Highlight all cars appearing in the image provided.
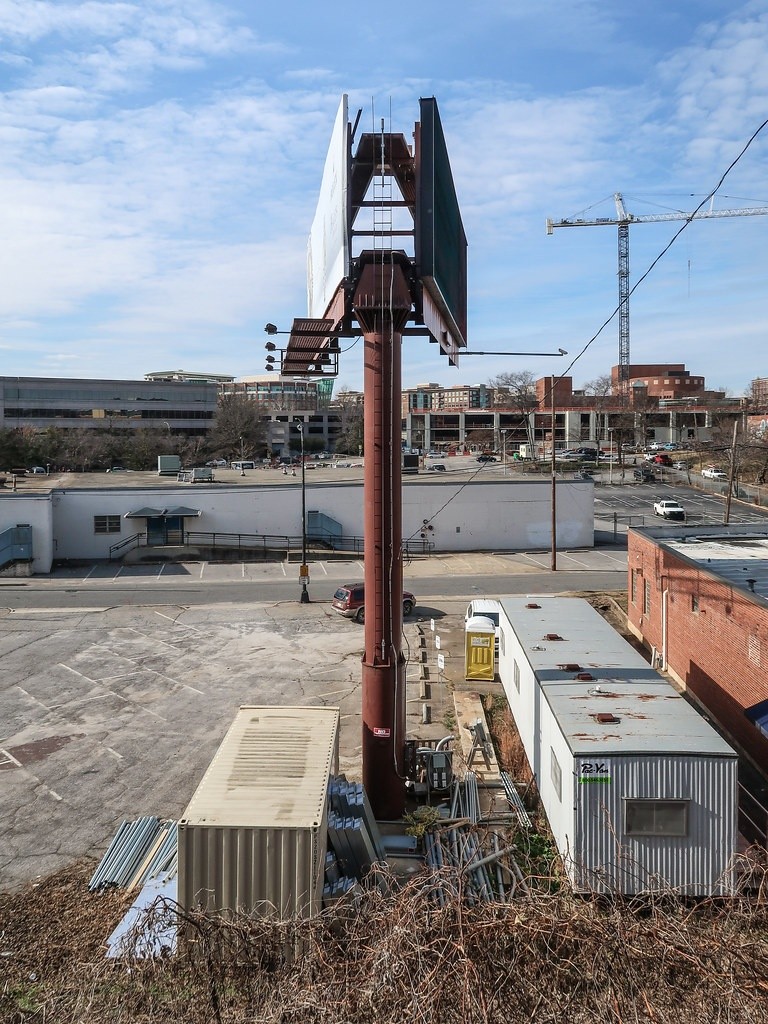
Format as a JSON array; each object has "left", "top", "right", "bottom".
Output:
[
  {"left": 428, "top": 464, "right": 446, "bottom": 471},
  {"left": 477, "top": 456, "right": 496, "bottom": 463},
  {"left": 206, "top": 457, "right": 226, "bottom": 466},
  {"left": 562, "top": 447, "right": 605, "bottom": 459}
]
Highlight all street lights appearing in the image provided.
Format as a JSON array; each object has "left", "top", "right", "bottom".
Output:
[
  {"left": 501, "top": 430, "right": 507, "bottom": 475},
  {"left": 608, "top": 427, "right": 615, "bottom": 484},
  {"left": 418, "top": 430, "right": 425, "bottom": 470},
  {"left": 240, "top": 436, "right": 245, "bottom": 476},
  {"left": 297, "top": 423, "right": 309, "bottom": 603}
]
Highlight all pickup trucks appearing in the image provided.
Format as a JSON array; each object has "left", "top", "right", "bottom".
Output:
[
  {"left": 664, "top": 442, "right": 678, "bottom": 451},
  {"left": 650, "top": 441, "right": 666, "bottom": 450},
  {"left": 655, "top": 454, "right": 672, "bottom": 465},
  {"left": 654, "top": 500, "right": 685, "bottom": 519},
  {"left": 701, "top": 467, "right": 727, "bottom": 481},
  {"left": 644, "top": 452, "right": 658, "bottom": 461}
]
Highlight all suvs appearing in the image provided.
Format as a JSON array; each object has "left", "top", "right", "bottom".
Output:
[
  {"left": 113, "top": 467, "right": 127, "bottom": 472},
  {"left": 620, "top": 442, "right": 631, "bottom": 451},
  {"left": 466, "top": 597, "right": 501, "bottom": 652},
  {"left": 332, "top": 582, "right": 416, "bottom": 624},
  {"left": 426, "top": 452, "right": 446, "bottom": 459},
  {"left": 581, "top": 466, "right": 593, "bottom": 475}
]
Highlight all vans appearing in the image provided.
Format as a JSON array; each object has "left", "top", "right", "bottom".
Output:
[
  {"left": 31, "top": 467, "right": 44, "bottom": 473},
  {"left": 673, "top": 461, "right": 686, "bottom": 470},
  {"left": 280, "top": 457, "right": 302, "bottom": 463}
]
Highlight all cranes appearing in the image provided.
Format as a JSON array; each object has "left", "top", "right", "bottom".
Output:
[{"left": 546, "top": 190, "right": 766, "bottom": 444}]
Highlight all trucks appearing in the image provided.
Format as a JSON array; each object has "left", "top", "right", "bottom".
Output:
[{"left": 634, "top": 469, "right": 655, "bottom": 482}]
